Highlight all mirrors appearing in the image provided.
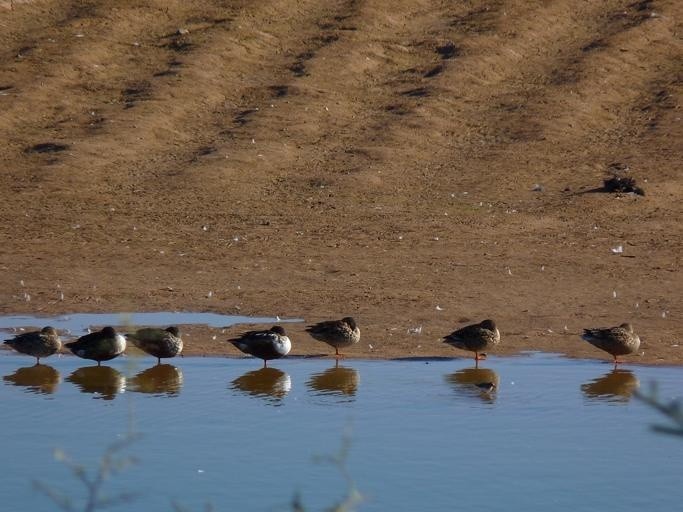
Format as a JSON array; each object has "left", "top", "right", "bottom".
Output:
[
  {"left": 305, "top": 316, "right": 361, "bottom": 356},
  {"left": 224, "top": 360, "right": 291, "bottom": 400},
  {"left": 60, "top": 325, "right": 127, "bottom": 365},
  {"left": 579, "top": 364, "right": 641, "bottom": 403},
  {"left": 226, "top": 324, "right": 293, "bottom": 360},
  {"left": 441, "top": 360, "right": 498, "bottom": 406},
  {"left": 127, "top": 362, "right": 189, "bottom": 398},
  {"left": 63, "top": 367, "right": 125, "bottom": 401},
  {"left": 1, "top": 324, "right": 62, "bottom": 362},
  {"left": 305, "top": 359, "right": 361, "bottom": 399},
  {"left": 2, "top": 364, "right": 60, "bottom": 396},
  {"left": 579, "top": 322, "right": 642, "bottom": 363},
  {"left": 441, "top": 318, "right": 502, "bottom": 361},
  {"left": 124, "top": 325, "right": 184, "bottom": 362}
]
